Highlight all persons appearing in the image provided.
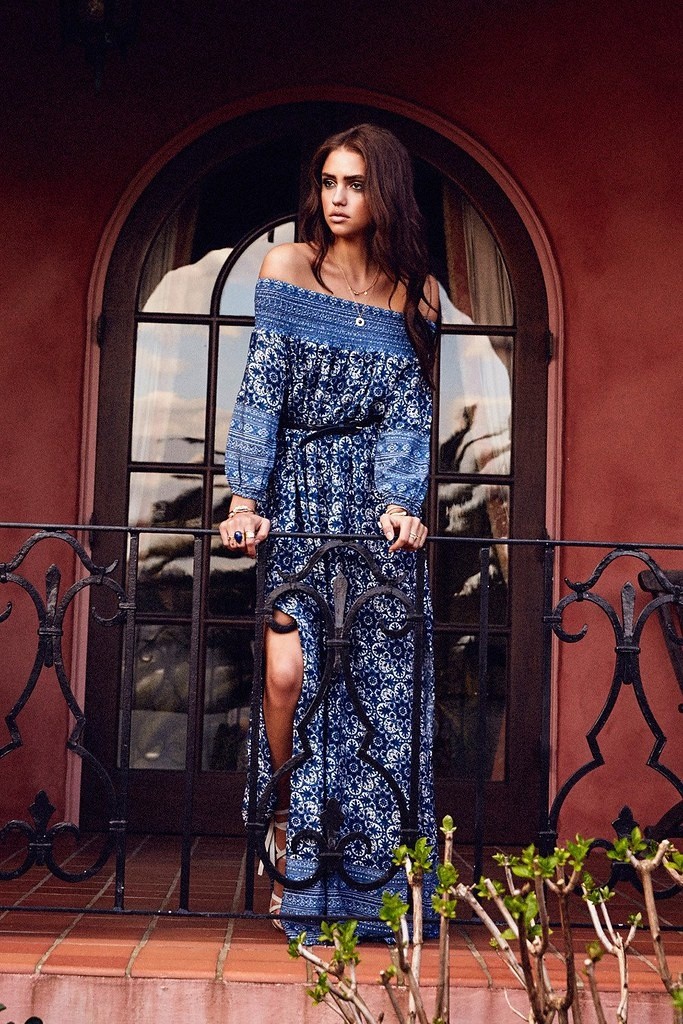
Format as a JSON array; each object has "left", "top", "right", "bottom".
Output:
[{"left": 220, "top": 123, "right": 439, "bottom": 943}]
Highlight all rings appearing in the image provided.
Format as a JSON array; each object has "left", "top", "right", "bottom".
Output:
[
  {"left": 228, "top": 536, "right": 234, "bottom": 540},
  {"left": 246, "top": 532, "right": 254, "bottom": 537},
  {"left": 410, "top": 533, "right": 418, "bottom": 540},
  {"left": 234, "top": 531, "right": 245, "bottom": 544}
]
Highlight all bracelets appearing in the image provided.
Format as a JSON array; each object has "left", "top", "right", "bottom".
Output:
[
  {"left": 386, "top": 507, "right": 410, "bottom": 516},
  {"left": 228, "top": 506, "right": 256, "bottom": 517}
]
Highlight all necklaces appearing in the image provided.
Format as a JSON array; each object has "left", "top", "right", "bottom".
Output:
[{"left": 333, "top": 255, "right": 373, "bottom": 326}]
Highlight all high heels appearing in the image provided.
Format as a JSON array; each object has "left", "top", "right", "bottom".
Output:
[{"left": 257, "top": 806, "right": 284, "bottom": 931}]
